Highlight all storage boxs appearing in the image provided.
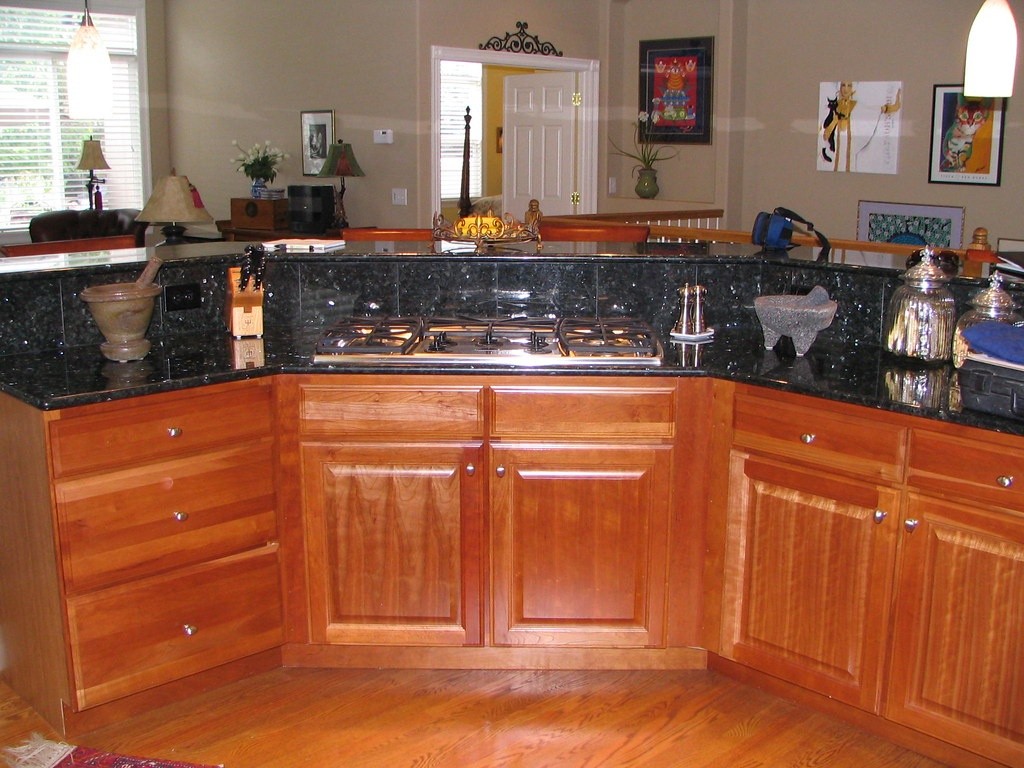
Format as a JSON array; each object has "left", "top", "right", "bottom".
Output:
[
  {"left": 645, "top": 239, "right": 712, "bottom": 258},
  {"left": 230, "top": 197, "right": 288, "bottom": 230}
]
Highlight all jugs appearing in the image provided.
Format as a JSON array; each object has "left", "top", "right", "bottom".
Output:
[{"left": 880, "top": 242, "right": 957, "bottom": 361}]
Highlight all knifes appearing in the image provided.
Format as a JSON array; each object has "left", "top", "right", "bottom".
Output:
[{"left": 235, "top": 243, "right": 266, "bottom": 294}]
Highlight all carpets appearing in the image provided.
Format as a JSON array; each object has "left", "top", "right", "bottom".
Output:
[{"left": 0, "top": 732, "right": 225, "bottom": 768}]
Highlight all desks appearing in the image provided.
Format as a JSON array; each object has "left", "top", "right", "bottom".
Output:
[{"left": 215, "top": 220, "right": 377, "bottom": 242}]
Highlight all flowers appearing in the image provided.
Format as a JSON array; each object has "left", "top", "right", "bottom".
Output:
[
  {"left": 607, "top": 97, "right": 681, "bottom": 178},
  {"left": 230, "top": 139, "right": 291, "bottom": 184}
]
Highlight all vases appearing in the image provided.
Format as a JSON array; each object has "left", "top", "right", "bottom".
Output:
[
  {"left": 634, "top": 169, "right": 659, "bottom": 199},
  {"left": 249, "top": 178, "right": 267, "bottom": 198}
]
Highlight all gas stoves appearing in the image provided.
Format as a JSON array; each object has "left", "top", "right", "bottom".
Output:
[{"left": 315, "top": 309, "right": 665, "bottom": 367}]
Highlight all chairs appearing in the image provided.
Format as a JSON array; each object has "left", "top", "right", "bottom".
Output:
[{"left": 30, "top": 208, "right": 141, "bottom": 242}]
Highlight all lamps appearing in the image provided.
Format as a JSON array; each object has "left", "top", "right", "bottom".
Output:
[
  {"left": 133, "top": 166, "right": 215, "bottom": 246},
  {"left": 314, "top": 138, "right": 367, "bottom": 200},
  {"left": 76, "top": 134, "right": 111, "bottom": 210},
  {"left": 66, "top": 0, "right": 115, "bottom": 121},
  {"left": 963, "top": 0, "right": 1018, "bottom": 100}
]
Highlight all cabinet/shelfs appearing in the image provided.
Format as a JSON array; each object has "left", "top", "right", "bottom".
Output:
[
  {"left": 0, "top": 375, "right": 290, "bottom": 739},
  {"left": 713, "top": 382, "right": 1024, "bottom": 768},
  {"left": 288, "top": 375, "right": 708, "bottom": 672}
]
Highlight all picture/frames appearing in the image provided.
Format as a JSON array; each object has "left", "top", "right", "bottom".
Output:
[
  {"left": 300, "top": 110, "right": 337, "bottom": 177},
  {"left": 997, "top": 236, "right": 1024, "bottom": 253},
  {"left": 856, "top": 200, "right": 966, "bottom": 250},
  {"left": 927, "top": 84, "right": 1008, "bottom": 188},
  {"left": 496, "top": 127, "right": 503, "bottom": 153},
  {"left": 637, "top": 35, "right": 714, "bottom": 147}
]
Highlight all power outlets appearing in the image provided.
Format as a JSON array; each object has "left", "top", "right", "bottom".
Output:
[
  {"left": 164, "top": 282, "right": 203, "bottom": 314},
  {"left": 392, "top": 188, "right": 408, "bottom": 206},
  {"left": 609, "top": 176, "right": 617, "bottom": 194}
]
